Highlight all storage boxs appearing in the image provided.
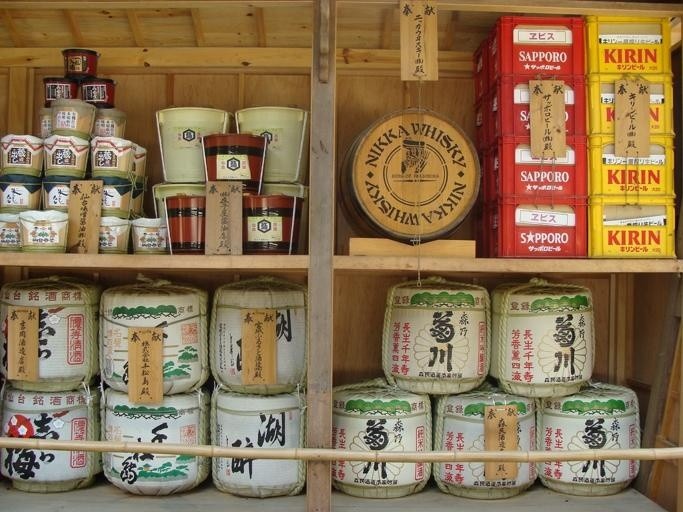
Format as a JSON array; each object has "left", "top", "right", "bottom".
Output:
[{"left": 467, "top": 14, "right": 675, "bottom": 261}]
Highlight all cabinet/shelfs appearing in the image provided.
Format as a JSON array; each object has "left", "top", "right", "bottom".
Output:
[{"left": 0, "top": 0, "right": 683, "bottom": 512}]
